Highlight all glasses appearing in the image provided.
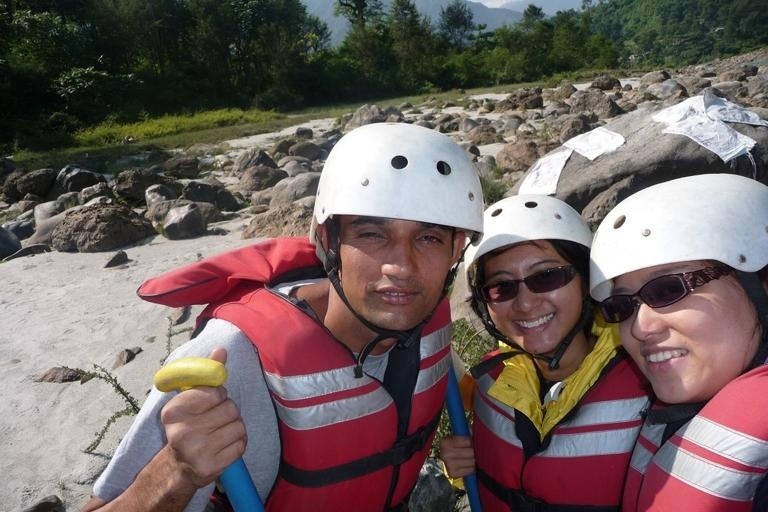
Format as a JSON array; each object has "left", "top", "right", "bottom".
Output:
[
  {"left": 596, "top": 262, "right": 734, "bottom": 324},
  {"left": 473, "top": 262, "right": 581, "bottom": 305}
]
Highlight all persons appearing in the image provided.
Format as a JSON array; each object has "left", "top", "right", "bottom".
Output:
[
  {"left": 81, "top": 123, "right": 484, "bottom": 512},
  {"left": 438, "top": 195, "right": 654, "bottom": 511},
  {"left": 588, "top": 173, "right": 768, "bottom": 511}
]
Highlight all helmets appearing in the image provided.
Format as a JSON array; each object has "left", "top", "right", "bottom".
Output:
[
  {"left": 460, "top": 192, "right": 596, "bottom": 296},
  {"left": 587, "top": 170, "right": 768, "bottom": 305},
  {"left": 306, "top": 119, "right": 486, "bottom": 254}
]
